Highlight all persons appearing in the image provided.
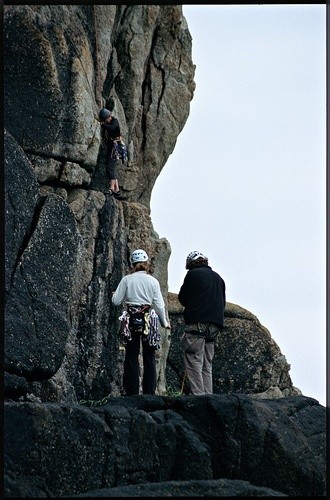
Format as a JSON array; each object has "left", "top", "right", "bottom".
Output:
[
  {"left": 112, "top": 249, "right": 172, "bottom": 397},
  {"left": 178, "top": 251, "right": 226, "bottom": 396}
]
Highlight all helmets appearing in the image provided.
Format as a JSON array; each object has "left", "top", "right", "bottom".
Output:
[
  {"left": 131, "top": 250, "right": 147, "bottom": 264},
  {"left": 186, "top": 251, "right": 206, "bottom": 262}
]
